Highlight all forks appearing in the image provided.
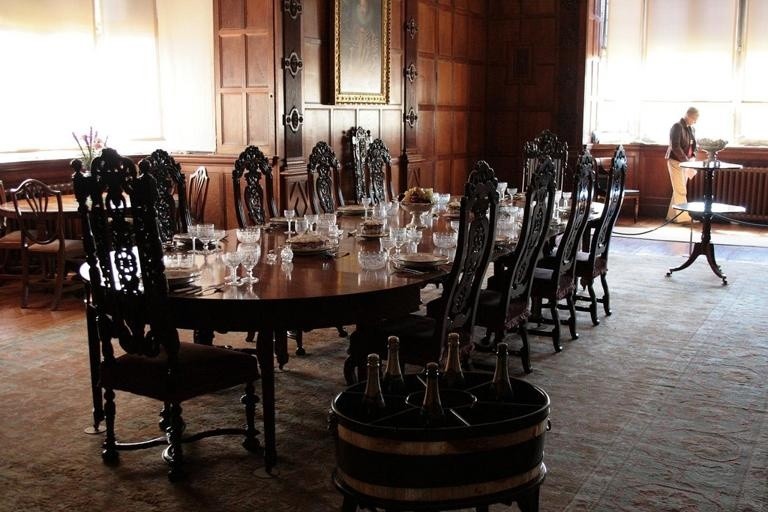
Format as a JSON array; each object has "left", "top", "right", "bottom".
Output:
[{"left": 195, "top": 284, "right": 226, "bottom": 296}]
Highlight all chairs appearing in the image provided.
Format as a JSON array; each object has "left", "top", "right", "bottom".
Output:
[
  {"left": 186, "top": 166, "right": 210, "bottom": 226},
  {"left": 344, "top": 161, "right": 500, "bottom": 388},
  {"left": 307, "top": 141, "right": 347, "bottom": 215},
  {"left": 0, "top": 180, "right": 48, "bottom": 294},
  {"left": 232, "top": 145, "right": 349, "bottom": 357},
  {"left": 426, "top": 155, "right": 556, "bottom": 375},
  {"left": 69, "top": 148, "right": 261, "bottom": 486},
  {"left": 527, "top": 144, "right": 627, "bottom": 327},
  {"left": 594, "top": 157, "right": 642, "bottom": 224},
  {"left": 10, "top": 178, "right": 84, "bottom": 311},
  {"left": 363, "top": 138, "right": 394, "bottom": 206},
  {"left": 135, "top": 148, "right": 271, "bottom": 371},
  {"left": 481, "top": 149, "right": 597, "bottom": 354}
]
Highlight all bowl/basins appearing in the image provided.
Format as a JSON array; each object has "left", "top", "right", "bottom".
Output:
[
  {"left": 236, "top": 228, "right": 260, "bottom": 243},
  {"left": 448, "top": 205, "right": 461, "bottom": 215},
  {"left": 361, "top": 224, "right": 383, "bottom": 235},
  {"left": 433, "top": 232, "right": 456, "bottom": 249}
]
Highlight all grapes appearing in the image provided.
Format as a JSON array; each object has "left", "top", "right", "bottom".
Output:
[{"left": 401, "top": 187, "right": 434, "bottom": 204}]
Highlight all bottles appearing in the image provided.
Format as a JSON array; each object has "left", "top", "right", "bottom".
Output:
[
  {"left": 383, "top": 336, "right": 408, "bottom": 397},
  {"left": 420, "top": 363, "right": 445, "bottom": 427},
  {"left": 488, "top": 343, "right": 513, "bottom": 403},
  {"left": 360, "top": 355, "right": 387, "bottom": 422},
  {"left": 443, "top": 333, "right": 465, "bottom": 390}
]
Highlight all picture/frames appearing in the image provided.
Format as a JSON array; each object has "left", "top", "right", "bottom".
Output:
[{"left": 329, "top": 0, "right": 392, "bottom": 106}]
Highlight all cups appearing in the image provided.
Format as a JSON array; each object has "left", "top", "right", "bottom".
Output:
[
  {"left": 324, "top": 213, "right": 336, "bottom": 225},
  {"left": 174, "top": 234, "right": 196, "bottom": 268},
  {"left": 380, "top": 232, "right": 396, "bottom": 258},
  {"left": 497, "top": 182, "right": 508, "bottom": 199},
  {"left": 222, "top": 252, "right": 242, "bottom": 286},
  {"left": 303, "top": 214, "right": 318, "bottom": 231},
  {"left": 197, "top": 223, "right": 214, "bottom": 254},
  {"left": 187, "top": 225, "right": 198, "bottom": 253},
  {"left": 562, "top": 192, "right": 572, "bottom": 207},
  {"left": 390, "top": 227, "right": 407, "bottom": 258},
  {"left": 213, "top": 230, "right": 225, "bottom": 253},
  {"left": 554, "top": 190, "right": 561, "bottom": 208},
  {"left": 388, "top": 203, "right": 399, "bottom": 215},
  {"left": 284, "top": 209, "right": 295, "bottom": 233},
  {"left": 451, "top": 220, "right": 460, "bottom": 233},
  {"left": 438, "top": 193, "right": 450, "bottom": 208},
  {"left": 295, "top": 219, "right": 308, "bottom": 235},
  {"left": 507, "top": 188, "right": 517, "bottom": 200},
  {"left": 237, "top": 242, "right": 259, "bottom": 284},
  {"left": 430, "top": 192, "right": 439, "bottom": 205},
  {"left": 362, "top": 198, "right": 372, "bottom": 218},
  {"left": 408, "top": 231, "right": 422, "bottom": 253},
  {"left": 373, "top": 206, "right": 385, "bottom": 218},
  {"left": 496, "top": 207, "right": 524, "bottom": 238}
]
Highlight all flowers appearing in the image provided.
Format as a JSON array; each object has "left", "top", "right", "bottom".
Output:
[{"left": 71, "top": 126, "right": 109, "bottom": 171}]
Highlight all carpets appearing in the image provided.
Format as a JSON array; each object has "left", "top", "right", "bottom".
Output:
[{"left": 0, "top": 234, "right": 768, "bottom": 512}]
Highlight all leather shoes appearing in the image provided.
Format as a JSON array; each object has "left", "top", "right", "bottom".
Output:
[
  {"left": 682, "top": 219, "right": 701, "bottom": 224},
  {"left": 666, "top": 216, "right": 676, "bottom": 221}
]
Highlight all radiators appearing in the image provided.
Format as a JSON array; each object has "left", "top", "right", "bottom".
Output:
[{"left": 686, "top": 166, "right": 768, "bottom": 223}]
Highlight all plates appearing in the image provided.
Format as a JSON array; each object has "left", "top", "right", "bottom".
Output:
[
  {"left": 399, "top": 254, "right": 440, "bottom": 262},
  {"left": 358, "top": 234, "right": 388, "bottom": 238},
  {"left": 443, "top": 214, "right": 460, "bottom": 219},
  {"left": 284, "top": 240, "right": 331, "bottom": 255},
  {"left": 165, "top": 267, "right": 198, "bottom": 279}
]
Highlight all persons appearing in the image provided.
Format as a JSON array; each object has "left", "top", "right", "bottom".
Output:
[{"left": 665, "top": 107, "right": 700, "bottom": 224}]
